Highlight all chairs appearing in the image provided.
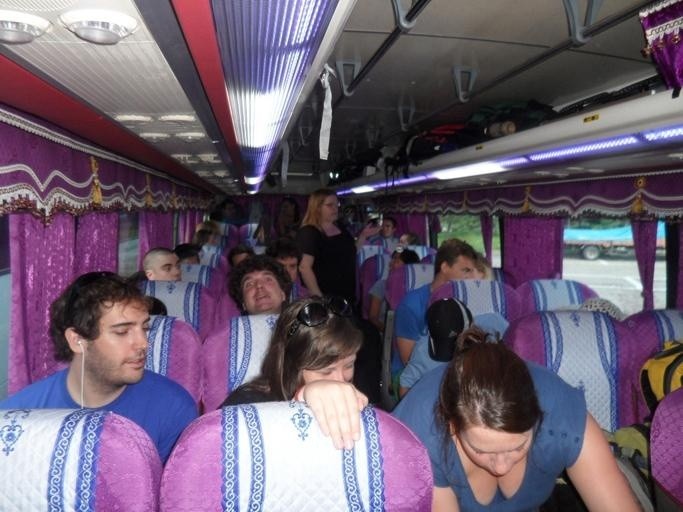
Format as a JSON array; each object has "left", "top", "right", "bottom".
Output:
[
  {"left": 1, "top": 408, "right": 161, "bottom": 511},
  {"left": 501, "top": 311, "right": 636, "bottom": 433},
  {"left": 157, "top": 402, "right": 433, "bottom": 512},
  {"left": 198, "top": 314, "right": 281, "bottom": 412},
  {"left": 647, "top": 389, "right": 682, "bottom": 512},
  {"left": 130, "top": 221, "right": 275, "bottom": 336},
  {"left": 351, "top": 242, "right": 602, "bottom": 407},
  {"left": 139, "top": 316, "right": 204, "bottom": 418},
  {"left": 619, "top": 306, "right": 683, "bottom": 424}
]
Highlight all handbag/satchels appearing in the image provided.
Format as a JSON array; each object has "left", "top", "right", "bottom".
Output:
[{"left": 638, "top": 340, "right": 682, "bottom": 414}]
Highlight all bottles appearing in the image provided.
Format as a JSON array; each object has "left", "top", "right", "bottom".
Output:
[{"left": 481, "top": 119, "right": 518, "bottom": 139}]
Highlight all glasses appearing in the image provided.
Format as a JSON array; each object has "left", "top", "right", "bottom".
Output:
[{"left": 287, "top": 294, "right": 353, "bottom": 340}]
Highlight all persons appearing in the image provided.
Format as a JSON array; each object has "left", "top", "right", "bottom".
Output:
[
  {"left": 215, "top": 295, "right": 369, "bottom": 448},
  {"left": 388, "top": 326, "right": 643, "bottom": 512},
  {"left": 0, "top": 271, "right": 196, "bottom": 466},
  {"left": 398, "top": 299, "right": 509, "bottom": 398},
  {"left": 142, "top": 188, "right": 494, "bottom": 318},
  {"left": 609, "top": 341, "right": 683, "bottom": 467},
  {"left": 391, "top": 239, "right": 477, "bottom": 403}
]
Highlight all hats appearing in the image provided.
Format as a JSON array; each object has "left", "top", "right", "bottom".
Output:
[{"left": 426, "top": 297, "right": 473, "bottom": 362}]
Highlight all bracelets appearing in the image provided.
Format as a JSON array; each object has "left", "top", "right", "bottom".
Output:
[{"left": 293, "top": 386, "right": 302, "bottom": 399}]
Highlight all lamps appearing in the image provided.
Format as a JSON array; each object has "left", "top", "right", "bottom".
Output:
[
  {"left": 335, "top": 126, "right": 683, "bottom": 197},
  {"left": 0, "top": 10, "right": 50, "bottom": 44},
  {"left": 116, "top": 113, "right": 242, "bottom": 201},
  {"left": 214, "top": 0, "right": 337, "bottom": 197},
  {"left": 61, "top": 10, "right": 139, "bottom": 46}
]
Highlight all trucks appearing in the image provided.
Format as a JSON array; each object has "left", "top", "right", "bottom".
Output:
[{"left": 562, "top": 221, "right": 665, "bottom": 261}]
